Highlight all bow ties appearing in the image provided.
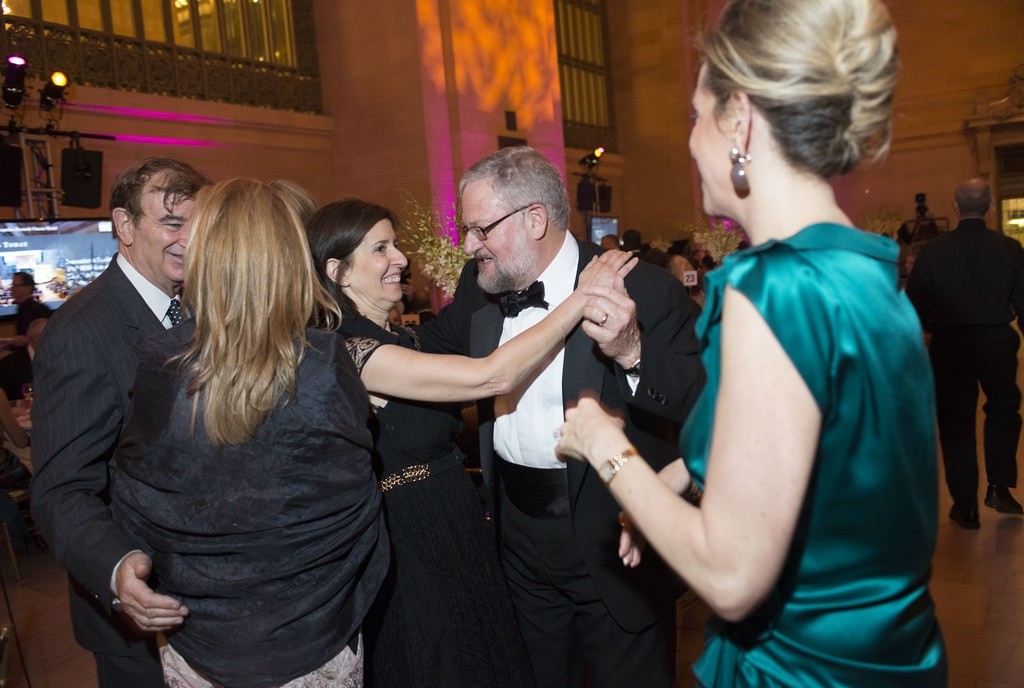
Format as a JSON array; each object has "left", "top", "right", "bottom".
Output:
[{"left": 498, "top": 281, "right": 549, "bottom": 318}]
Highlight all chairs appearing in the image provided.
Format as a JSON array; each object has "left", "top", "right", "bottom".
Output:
[{"left": 2, "top": 489, "right": 45, "bottom": 582}]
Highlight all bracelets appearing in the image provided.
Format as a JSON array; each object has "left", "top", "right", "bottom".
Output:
[{"left": 548, "top": 313, "right": 566, "bottom": 347}]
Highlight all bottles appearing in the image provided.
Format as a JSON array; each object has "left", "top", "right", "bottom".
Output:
[
  {"left": 400, "top": 259, "right": 411, "bottom": 303},
  {"left": 24, "top": 382, "right": 34, "bottom": 407}
]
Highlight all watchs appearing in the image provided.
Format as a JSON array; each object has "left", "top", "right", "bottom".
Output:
[
  {"left": 622, "top": 358, "right": 641, "bottom": 377},
  {"left": 598, "top": 446, "right": 640, "bottom": 490}
]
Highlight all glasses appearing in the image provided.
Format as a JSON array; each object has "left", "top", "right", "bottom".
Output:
[{"left": 460, "top": 203, "right": 529, "bottom": 241}]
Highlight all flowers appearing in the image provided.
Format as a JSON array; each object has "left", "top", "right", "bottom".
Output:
[
  {"left": 865, "top": 215, "right": 902, "bottom": 240},
  {"left": 397, "top": 189, "right": 474, "bottom": 300},
  {"left": 679, "top": 218, "right": 743, "bottom": 263},
  {"left": 649, "top": 237, "right": 672, "bottom": 253}
]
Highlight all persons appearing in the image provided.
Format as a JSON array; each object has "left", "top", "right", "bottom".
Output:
[
  {"left": 0, "top": 271, "right": 53, "bottom": 564},
  {"left": 906, "top": 176, "right": 1024, "bottom": 530},
  {"left": 553, "top": 0, "right": 950, "bottom": 688},
  {"left": 600, "top": 230, "right": 717, "bottom": 315},
  {"left": 398, "top": 146, "right": 709, "bottom": 688},
  {"left": 32, "top": 157, "right": 212, "bottom": 688},
  {"left": 399, "top": 277, "right": 415, "bottom": 315},
  {"left": 303, "top": 201, "right": 639, "bottom": 688},
  {"left": 108, "top": 176, "right": 389, "bottom": 688}
]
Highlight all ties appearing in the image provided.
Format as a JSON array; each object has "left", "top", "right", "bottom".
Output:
[{"left": 167, "top": 299, "right": 182, "bottom": 327}]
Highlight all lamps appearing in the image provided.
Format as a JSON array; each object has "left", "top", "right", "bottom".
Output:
[
  {"left": 39, "top": 72, "right": 69, "bottom": 111},
  {"left": 3, "top": 52, "right": 25, "bottom": 110},
  {"left": 577, "top": 147, "right": 605, "bottom": 170}
]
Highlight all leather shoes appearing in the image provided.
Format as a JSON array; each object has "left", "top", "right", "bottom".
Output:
[
  {"left": 949, "top": 503, "right": 980, "bottom": 529},
  {"left": 984, "top": 484, "right": 1023, "bottom": 513}
]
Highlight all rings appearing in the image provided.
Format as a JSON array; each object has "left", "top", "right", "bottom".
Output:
[{"left": 599, "top": 313, "right": 609, "bottom": 326}]
